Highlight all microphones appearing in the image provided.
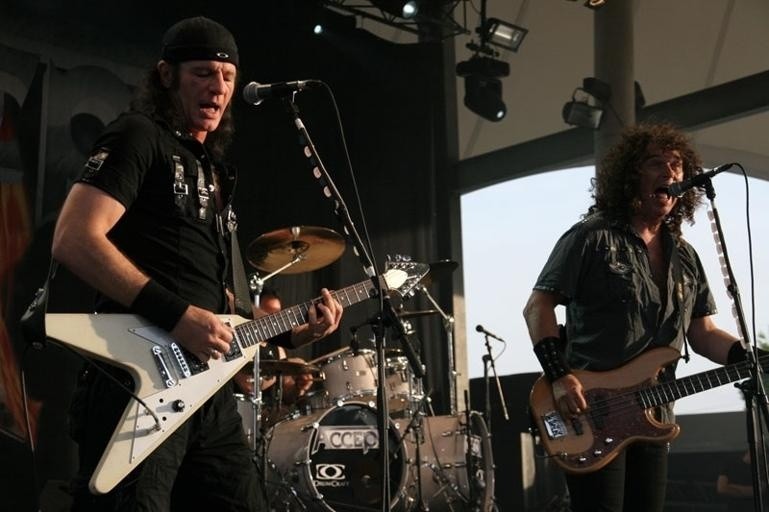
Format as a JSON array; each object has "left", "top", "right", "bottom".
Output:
[
  {"left": 243, "top": 79, "right": 320, "bottom": 104},
  {"left": 476, "top": 326, "right": 502, "bottom": 342},
  {"left": 668, "top": 164, "right": 731, "bottom": 197}
]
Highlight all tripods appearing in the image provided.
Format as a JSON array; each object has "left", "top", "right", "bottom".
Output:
[
  {"left": 397, "top": 320, "right": 478, "bottom": 512},
  {"left": 247, "top": 258, "right": 308, "bottom": 512}
]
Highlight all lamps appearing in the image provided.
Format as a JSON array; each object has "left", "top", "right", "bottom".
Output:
[
  {"left": 461, "top": 13, "right": 529, "bottom": 126},
  {"left": 370, "top": 0, "right": 419, "bottom": 19}
]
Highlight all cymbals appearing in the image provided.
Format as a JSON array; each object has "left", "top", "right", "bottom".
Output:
[
  {"left": 242, "top": 360, "right": 319, "bottom": 375},
  {"left": 248, "top": 227, "right": 346, "bottom": 276}
]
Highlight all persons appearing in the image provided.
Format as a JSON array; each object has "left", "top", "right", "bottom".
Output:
[
  {"left": 523, "top": 120, "right": 769, "bottom": 512},
  {"left": 20, "top": 17, "right": 343, "bottom": 512}
]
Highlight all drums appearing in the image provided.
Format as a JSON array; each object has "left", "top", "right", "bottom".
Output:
[
  {"left": 385, "top": 350, "right": 424, "bottom": 416},
  {"left": 258, "top": 401, "right": 408, "bottom": 511},
  {"left": 319, "top": 349, "right": 378, "bottom": 406},
  {"left": 394, "top": 412, "right": 494, "bottom": 511}
]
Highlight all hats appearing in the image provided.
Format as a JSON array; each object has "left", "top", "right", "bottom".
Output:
[{"left": 160, "top": 15, "right": 242, "bottom": 70}]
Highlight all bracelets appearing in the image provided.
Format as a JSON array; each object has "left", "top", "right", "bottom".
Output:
[
  {"left": 728, "top": 340, "right": 769, "bottom": 374},
  {"left": 533, "top": 336, "right": 571, "bottom": 383}
]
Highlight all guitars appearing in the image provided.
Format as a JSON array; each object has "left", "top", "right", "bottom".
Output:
[
  {"left": 529, "top": 345, "right": 769, "bottom": 473},
  {"left": 44, "top": 254, "right": 431, "bottom": 493}
]
